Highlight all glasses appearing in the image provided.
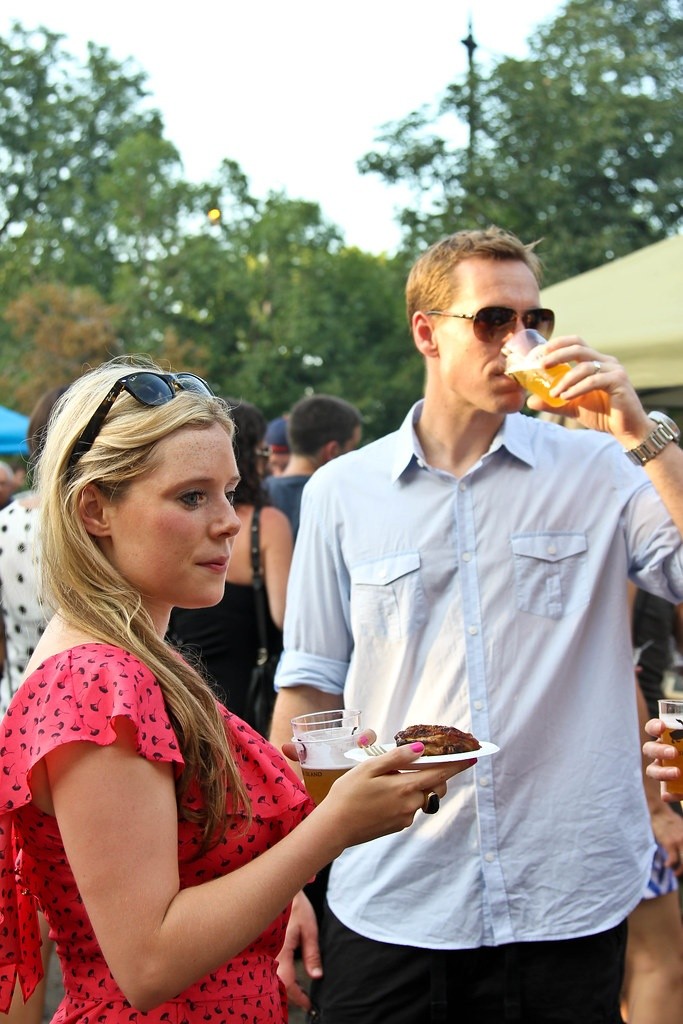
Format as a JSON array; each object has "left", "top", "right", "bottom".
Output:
[
  {"left": 426, "top": 306, "right": 555, "bottom": 347},
  {"left": 63, "top": 372, "right": 214, "bottom": 481}
]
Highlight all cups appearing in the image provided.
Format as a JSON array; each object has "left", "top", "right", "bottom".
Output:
[
  {"left": 289, "top": 708, "right": 364, "bottom": 807},
  {"left": 658, "top": 700, "right": 683, "bottom": 796},
  {"left": 500, "top": 329, "right": 574, "bottom": 407}
]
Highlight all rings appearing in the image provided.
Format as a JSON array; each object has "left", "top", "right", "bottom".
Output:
[{"left": 422, "top": 788, "right": 440, "bottom": 814}]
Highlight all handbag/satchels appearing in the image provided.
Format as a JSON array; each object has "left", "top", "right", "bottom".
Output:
[{"left": 250, "top": 655, "right": 276, "bottom": 734}]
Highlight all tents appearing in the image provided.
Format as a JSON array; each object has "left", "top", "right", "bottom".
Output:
[{"left": 540, "top": 229, "right": 683, "bottom": 395}]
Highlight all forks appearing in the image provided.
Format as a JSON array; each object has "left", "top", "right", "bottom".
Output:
[{"left": 361, "top": 744, "right": 388, "bottom": 758}]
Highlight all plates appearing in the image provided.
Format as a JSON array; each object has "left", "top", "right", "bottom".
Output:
[{"left": 344, "top": 740, "right": 500, "bottom": 769}]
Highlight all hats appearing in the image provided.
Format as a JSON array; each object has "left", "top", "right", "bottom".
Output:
[{"left": 266, "top": 419, "right": 288, "bottom": 452}]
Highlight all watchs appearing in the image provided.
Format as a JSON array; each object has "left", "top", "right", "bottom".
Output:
[{"left": 623, "top": 411, "right": 681, "bottom": 467}]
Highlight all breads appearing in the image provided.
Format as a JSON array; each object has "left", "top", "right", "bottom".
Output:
[{"left": 396, "top": 726, "right": 480, "bottom": 757}]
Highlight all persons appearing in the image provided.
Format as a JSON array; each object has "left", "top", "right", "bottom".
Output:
[
  {"left": 163, "top": 394, "right": 362, "bottom": 735},
  {"left": 0, "top": 363, "right": 478, "bottom": 1024},
  {"left": 0, "top": 383, "right": 68, "bottom": 722},
  {"left": 269, "top": 226, "right": 683, "bottom": 1024},
  {"left": 623, "top": 582, "right": 683, "bottom": 1024}
]
